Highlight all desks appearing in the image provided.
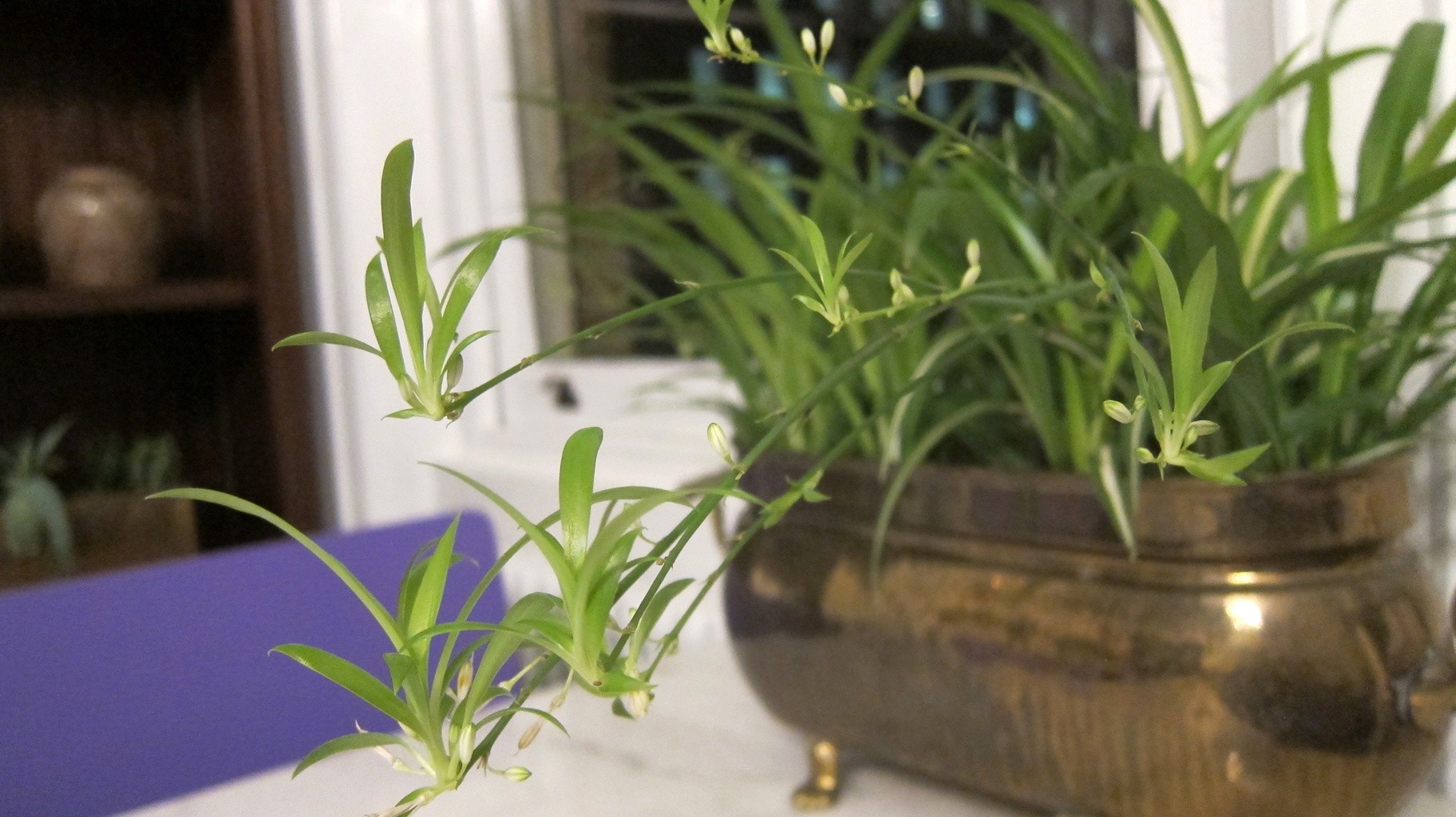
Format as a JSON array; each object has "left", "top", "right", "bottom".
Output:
[{"left": 123, "top": 623, "right": 1456, "bottom": 817}]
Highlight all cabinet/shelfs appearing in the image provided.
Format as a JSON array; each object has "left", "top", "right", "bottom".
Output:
[{"left": 0, "top": 0, "right": 335, "bottom": 589}]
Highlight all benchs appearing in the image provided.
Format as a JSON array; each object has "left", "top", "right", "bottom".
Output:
[{"left": 0, "top": 508, "right": 525, "bottom": 816}]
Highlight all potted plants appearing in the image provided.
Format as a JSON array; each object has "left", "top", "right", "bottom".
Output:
[{"left": 317, "top": 0, "right": 1456, "bottom": 817}]
